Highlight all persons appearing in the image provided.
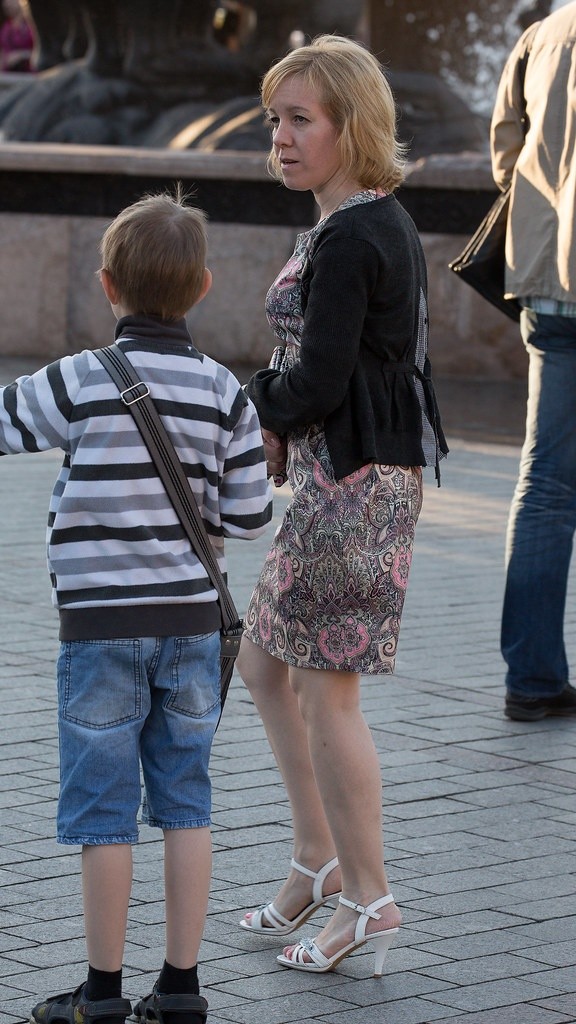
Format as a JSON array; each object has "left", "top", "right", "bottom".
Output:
[
  {"left": 491, "top": 3, "right": 576, "bottom": 720},
  {"left": 214, "top": 0, "right": 255, "bottom": 50},
  {"left": 0, "top": 193, "right": 273, "bottom": 1024},
  {"left": 0, "top": 0, "right": 47, "bottom": 73},
  {"left": 242, "top": 36, "right": 431, "bottom": 978}
]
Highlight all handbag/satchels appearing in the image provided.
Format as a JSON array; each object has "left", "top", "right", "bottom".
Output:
[
  {"left": 218, "top": 619, "right": 242, "bottom": 731},
  {"left": 449, "top": 183, "right": 520, "bottom": 321}
]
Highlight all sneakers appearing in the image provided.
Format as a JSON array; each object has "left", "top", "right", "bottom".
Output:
[{"left": 505, "top": 684, "right": 576, "bottom": 721}]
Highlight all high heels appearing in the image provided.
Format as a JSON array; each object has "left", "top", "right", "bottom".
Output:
[
  {"left": 239, "top": 856, "right": 343, "bottom": 935},
  {"left": 276, "top": 894, "right": 401, "bottom": 979}
]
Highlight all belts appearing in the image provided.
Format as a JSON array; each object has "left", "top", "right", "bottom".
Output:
[{"left": 527, "top": 301, "right": 576, "bottom": 318}]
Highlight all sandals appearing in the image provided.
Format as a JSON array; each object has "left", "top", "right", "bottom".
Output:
[
  {"left": 33, "top": 981, "right": 131, "bottom": 1024},
  {"left": 132, "top": 980, "right": 208, "bottom": 1024}
]
